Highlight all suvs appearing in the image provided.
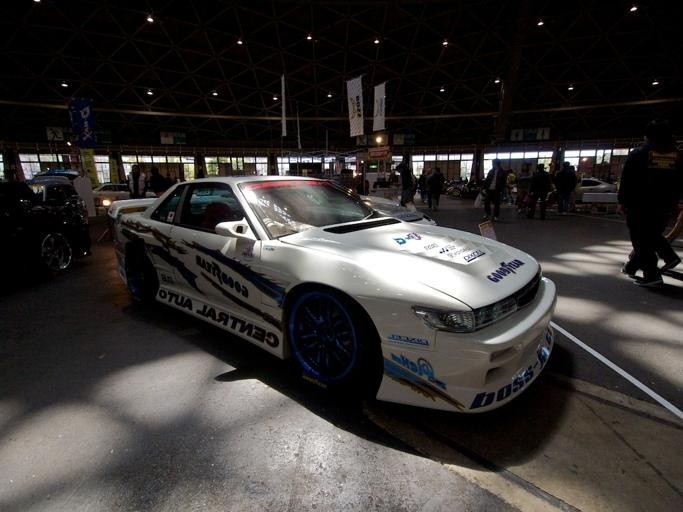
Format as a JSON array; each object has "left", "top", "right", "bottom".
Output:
[{"left": 0, "top": 175, "right": 93, "bottom": 273}]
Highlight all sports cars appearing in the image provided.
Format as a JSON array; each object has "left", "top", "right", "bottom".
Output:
[{"left": 104, "top": 172, "right": 560, "bottom": 418}]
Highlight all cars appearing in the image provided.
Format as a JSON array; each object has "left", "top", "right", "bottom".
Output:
[
  {"left": 93, "top": 183, "right": 156, "bottom": 207},
  {"left": 547, "top": 177, "right": 617, "bottom": 204}
]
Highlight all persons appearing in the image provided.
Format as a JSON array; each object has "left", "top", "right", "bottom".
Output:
[
  {"left": 617, "top": 117, "right": 682, "bottom": 290},
  {"left": 126, "top": 164, "right": 148, "bottom": 200},
  {"left": 164, "top": 173, "right": 187, "bottom": 189},
  {"left": 196, "top": 166, "right": 204, "bottom": 179},
  {"left": 395, "top": 152, "right": 578, "bottom": 224},
  {"left": 627, "top": 204, "right": 682, "bottom": 274},
  {"left": 148, "top": 165, "right": 168, "bottom": 198}
]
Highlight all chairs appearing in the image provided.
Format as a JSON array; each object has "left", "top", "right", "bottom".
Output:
[{"left": 201, "top": 202, "right": 235, "bottom": 230}]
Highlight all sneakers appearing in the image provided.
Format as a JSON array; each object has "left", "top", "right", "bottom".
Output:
[
  {"left": 621, "top": 263, "right": 636, "bottom": 277},
  {"left": 632, "top": 277, "right": 662, "bottom": 286},
  {"left": 660, "top": 259, "right": 681, "bottom": 271}
]
